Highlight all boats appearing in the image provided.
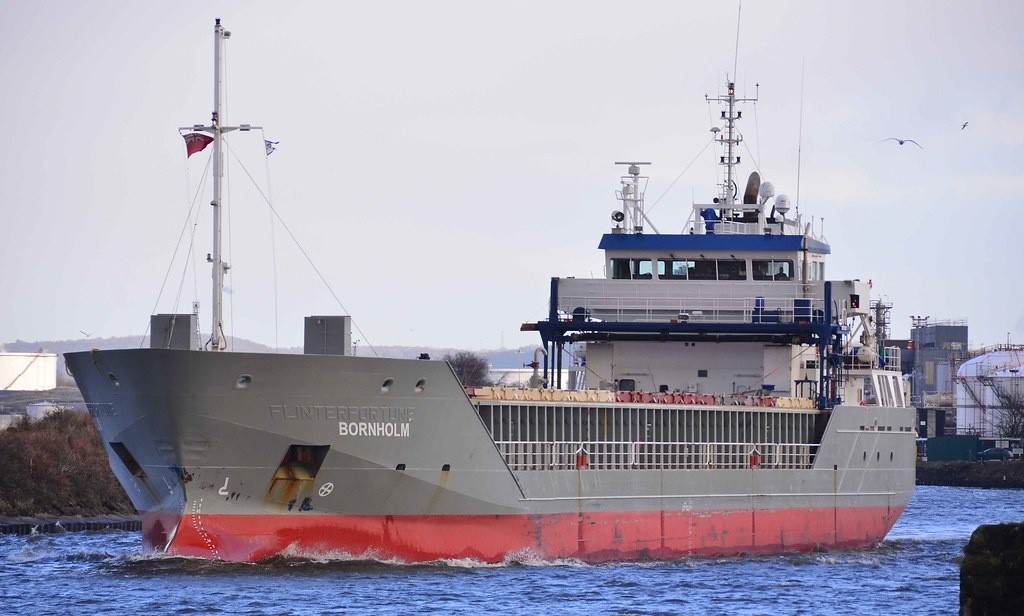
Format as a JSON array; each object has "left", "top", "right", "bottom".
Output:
[{"left": 62, "top": 17, "right": 930, "bottom": 569}]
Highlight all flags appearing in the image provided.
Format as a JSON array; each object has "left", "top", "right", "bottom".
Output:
[
  {"left": 264, "top": 141, "right": 279, "bottom": 155},
  {"left": 183, "top": 133, "right": 214, "bottom": 158}
]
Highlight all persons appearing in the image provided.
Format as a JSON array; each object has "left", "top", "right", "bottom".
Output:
[{"left": 774, "top": 267, "right": 787, "bottom": 277}]
